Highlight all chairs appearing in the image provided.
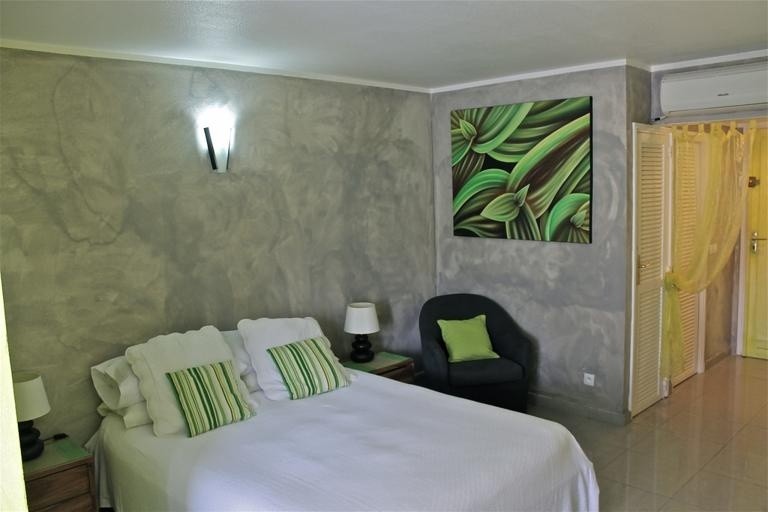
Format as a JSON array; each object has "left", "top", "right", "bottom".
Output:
[{"left": 418, "top": 292, "right": 533, "bottom": 413}]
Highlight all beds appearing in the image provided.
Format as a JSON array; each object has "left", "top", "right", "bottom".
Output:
[{"left": 95, "top": 362, "right": 588, "bottom": 512}]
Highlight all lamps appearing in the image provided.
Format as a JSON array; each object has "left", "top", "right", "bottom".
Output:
[
  {"left": 204, "top": 123, "right": 233, "bottom": 172},
  {"left": 12, "top": 372, "right": 51, "bottom": 463},
  {"left": 343, "top": 301, "right": 381, "bottom": 363}
]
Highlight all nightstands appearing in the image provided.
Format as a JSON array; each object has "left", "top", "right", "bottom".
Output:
[
  {"left": 343, "top": 350, "right": 415, "bottom": 384},
  {"left": 20, "top": 437, "right": 95, "bottom": 511}
]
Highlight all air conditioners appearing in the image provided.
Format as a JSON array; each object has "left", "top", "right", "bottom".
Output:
[{"left": 658, "top": 58, "right": 767, "bottom": 119}]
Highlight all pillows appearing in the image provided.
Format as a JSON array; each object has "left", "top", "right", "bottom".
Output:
[
  {"left": 130, "top": 315, "right": 354, "bottom": 437},
  {"left": 435, "top": 313, "right": 500, "bottom": 363}
]
